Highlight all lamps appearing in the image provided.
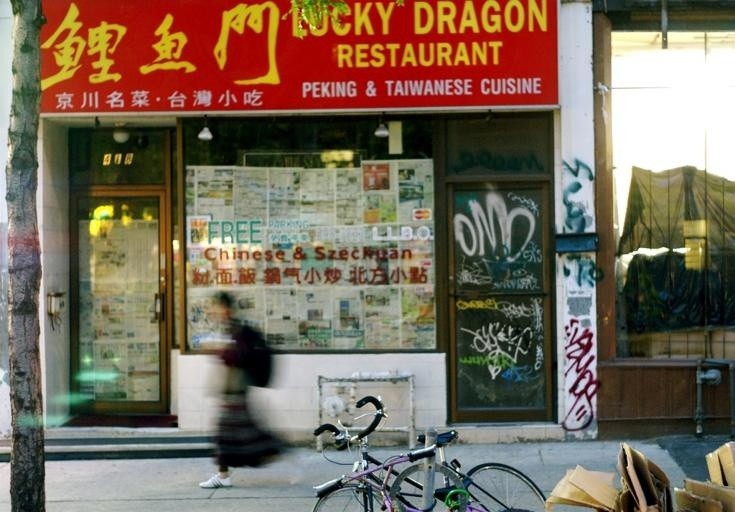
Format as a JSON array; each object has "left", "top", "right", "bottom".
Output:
[
  {"left": 198, "top": 113, "right": 213, "bottom": 141},
  {"left": 113, "top": 121, "right": 129, "bottom": 144},
  {"left": 374, "top": 111, "right": 390, "bottom": 138}
]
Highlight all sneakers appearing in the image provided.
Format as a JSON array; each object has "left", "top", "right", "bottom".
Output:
[{"left": 197, "top": 473, "right": 231, "bottom": 489}]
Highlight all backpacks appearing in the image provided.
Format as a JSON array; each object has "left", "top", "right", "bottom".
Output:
[{"left": 242, "top": 326, "right": 272, "bottom": 389}]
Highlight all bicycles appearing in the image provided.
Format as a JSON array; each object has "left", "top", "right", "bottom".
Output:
[{"left": 313, "top": 396, "right": 547, "bottom": 512}]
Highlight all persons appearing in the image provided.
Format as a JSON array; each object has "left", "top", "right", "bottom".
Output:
[{"left": 195, "top": 288, "right": 299, "bottom": 488}]
